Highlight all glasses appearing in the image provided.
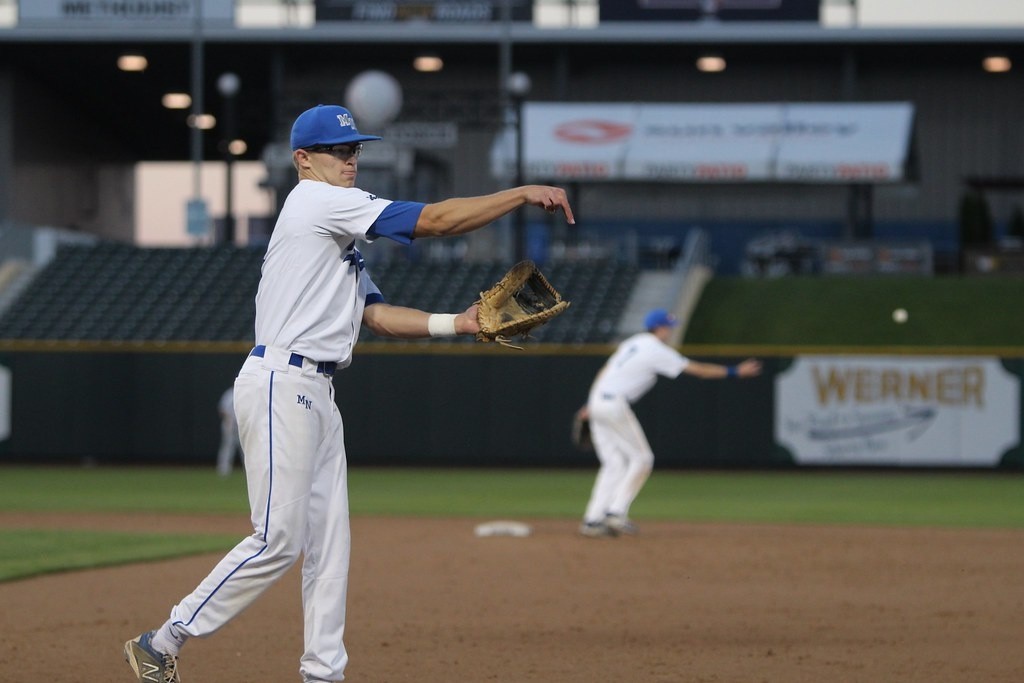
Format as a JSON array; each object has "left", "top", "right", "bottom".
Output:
[{"left": 304, "top": 142, "right": 364, "bottom": 161}]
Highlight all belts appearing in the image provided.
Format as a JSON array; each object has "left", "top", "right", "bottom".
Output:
[{"left": 250, "top": 345, "right": 337, "bottom": 377}]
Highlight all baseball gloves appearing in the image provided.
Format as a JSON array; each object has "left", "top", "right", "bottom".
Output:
[{"left": 474, "top": 256, "right": 574, "bottom": 338}]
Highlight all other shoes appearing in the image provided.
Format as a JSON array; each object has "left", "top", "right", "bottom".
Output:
[
  {"left": 580, "top": 523, "right": 607, "bottom": 539},
  {"left": 604, "top": 513, "right": 640, "bottom": 537}
]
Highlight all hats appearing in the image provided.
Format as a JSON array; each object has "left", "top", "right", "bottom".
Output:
[
  {"left": 291, "top": 104, "right": 383, "bottom": 151},
  {"left": 645, "top": 308, "right": 681, "bottom": 327}
]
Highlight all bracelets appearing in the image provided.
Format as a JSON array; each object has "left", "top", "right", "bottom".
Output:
[
  {"left": 727, "top": 365, "right": 737, "bottom": 378},
  {"left": 428, "top": 313, "right": 459, "bottom": 338}
]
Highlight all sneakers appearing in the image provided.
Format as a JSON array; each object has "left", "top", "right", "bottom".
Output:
[{"left": 123, "top": 629, "right": 182, "bottom": 683}]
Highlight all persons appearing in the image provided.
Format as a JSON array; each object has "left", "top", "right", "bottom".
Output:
[
  {"left": 123, "top": 104, "right": 576, "bottom": 683},
  {"left": 217, "top": 389, "right": 245, "bottom": 476},
  {"left": 578, "top": 308, "right": 760, "bottom": 534}
]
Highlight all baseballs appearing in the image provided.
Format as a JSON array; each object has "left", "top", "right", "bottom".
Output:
[{"left": 891, "top": 309, "right": 909, "bottom": 325}]
